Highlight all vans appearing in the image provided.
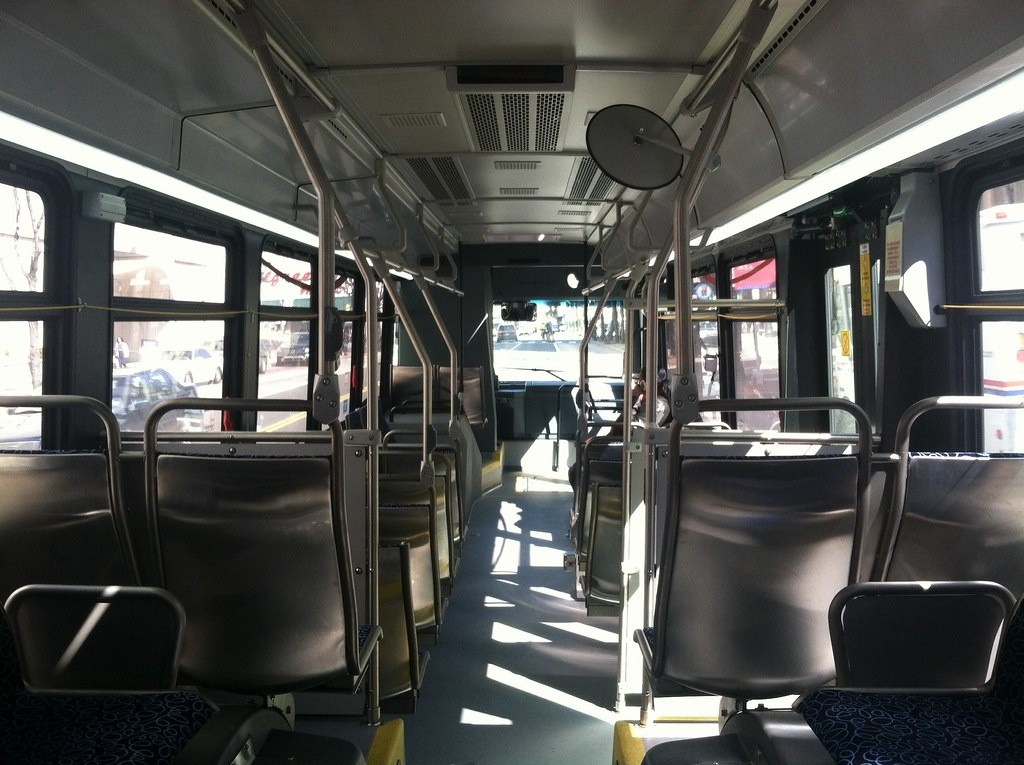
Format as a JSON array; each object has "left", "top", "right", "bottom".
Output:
[{"left": 980, "top": 201, "right": 1024, "bottom": 457}]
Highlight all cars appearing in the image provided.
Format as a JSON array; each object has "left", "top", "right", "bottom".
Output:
[
  {"left": 276, "top": 331, "right": 310, "bottom": 366},
  {"left": 0, "top": 365, "right": 203, "bottom": 451},
  {"left": 216, "top": 338, "right": 268, "bottom": 374},
  {"left": 139, "top": 338, "right": 223, "bottom": 388}
]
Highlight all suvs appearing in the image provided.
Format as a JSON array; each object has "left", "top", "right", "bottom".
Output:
[{"left": 497, "top": 325, "right": 517, "bottom": 341}]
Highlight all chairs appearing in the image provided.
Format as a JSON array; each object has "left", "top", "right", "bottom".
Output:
[
  {"left": 570, "top": 374, "right": 1024, "bottom": 765},
  {"left": 0, "top": 364, "right": 490, "bottom": 765}
]
